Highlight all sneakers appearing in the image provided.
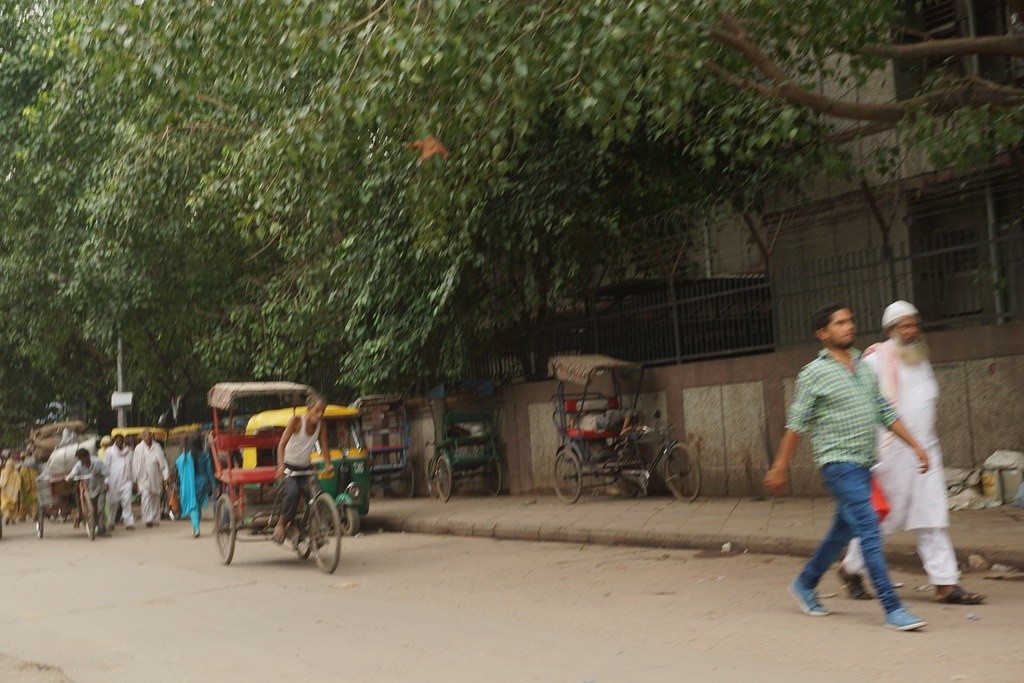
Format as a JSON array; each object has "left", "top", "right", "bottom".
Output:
[
  {"left": 791, "top": 577, "right": 830, "bottom": 615},
  {"left": 882, "top": 605, "right": 927, "bottom": 630}
]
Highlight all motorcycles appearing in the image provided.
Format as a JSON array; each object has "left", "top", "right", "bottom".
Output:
[{"left": 112, "top": 405, "right": 372, "bottom": 537}]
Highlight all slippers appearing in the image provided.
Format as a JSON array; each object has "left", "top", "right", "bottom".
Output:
[
  {"left": 933, "top": 586, "right": 985, "bottom": 603},
  {"left": 838, "top": 567, "right": 874, "bottom": 599}
]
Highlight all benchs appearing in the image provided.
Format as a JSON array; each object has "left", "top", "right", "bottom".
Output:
[
  {"left": 437, "top": 433, "right": 493, "bottom": 468},
  {"left": 361, "top": 426, "right": 406, "bottom": 470},
  {"left": 211, "top": 434, "right": 281, "bottom": 520},
  {"left": 550, "top": 395, "right": 625, "bottom": 437}
]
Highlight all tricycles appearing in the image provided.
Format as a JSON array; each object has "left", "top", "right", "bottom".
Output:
[
  {"left": 347, "top": 394, "right": 417, "bottom": 500},
  {"left": 547, "top": 354, "right": 701, "bottom": 505},
  {"left": 426, "top": 378, "right": 505, "bottom": 503},
  {"left": 208, "top": 381, "right": 342, "bottom": 573},
  {"left": 35, "top": 473, "right": 106, "bottom": 541}
]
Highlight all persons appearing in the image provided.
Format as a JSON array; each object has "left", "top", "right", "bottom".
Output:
[
  {"left": 762, "top": 304, "right": 928, "bottom": 631},
  {"left": 64, "top": 449, "right": 111, "bottom": 539},
  {"left": 272, "top": 392, "right": 334, "bottom": 545},
  {"left": 837, "top": 301, "right": 988, "bottom": 605},
  {"left": 174, "top": 430, "right": 233, "bottom": 537},
  {"left": 98, "top": 435, "right": 112, "bottom": 460},
  {"left": 103, "top": 434, "right": 135, "bottom": 531},
  {"left": 0, "top": 455, "right": 38, "bottom": 526},
  {"left": 132, "top": 430, "right": 171, "bottom": 527}
]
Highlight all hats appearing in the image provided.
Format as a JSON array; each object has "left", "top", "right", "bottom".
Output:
[{"left": 881, "top": 300, "right": 918, "bottom": 325}]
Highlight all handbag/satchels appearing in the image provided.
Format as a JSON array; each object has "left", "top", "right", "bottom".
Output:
[{"left": 871, "top": 477, "right": 890, "bottom": 524}]
